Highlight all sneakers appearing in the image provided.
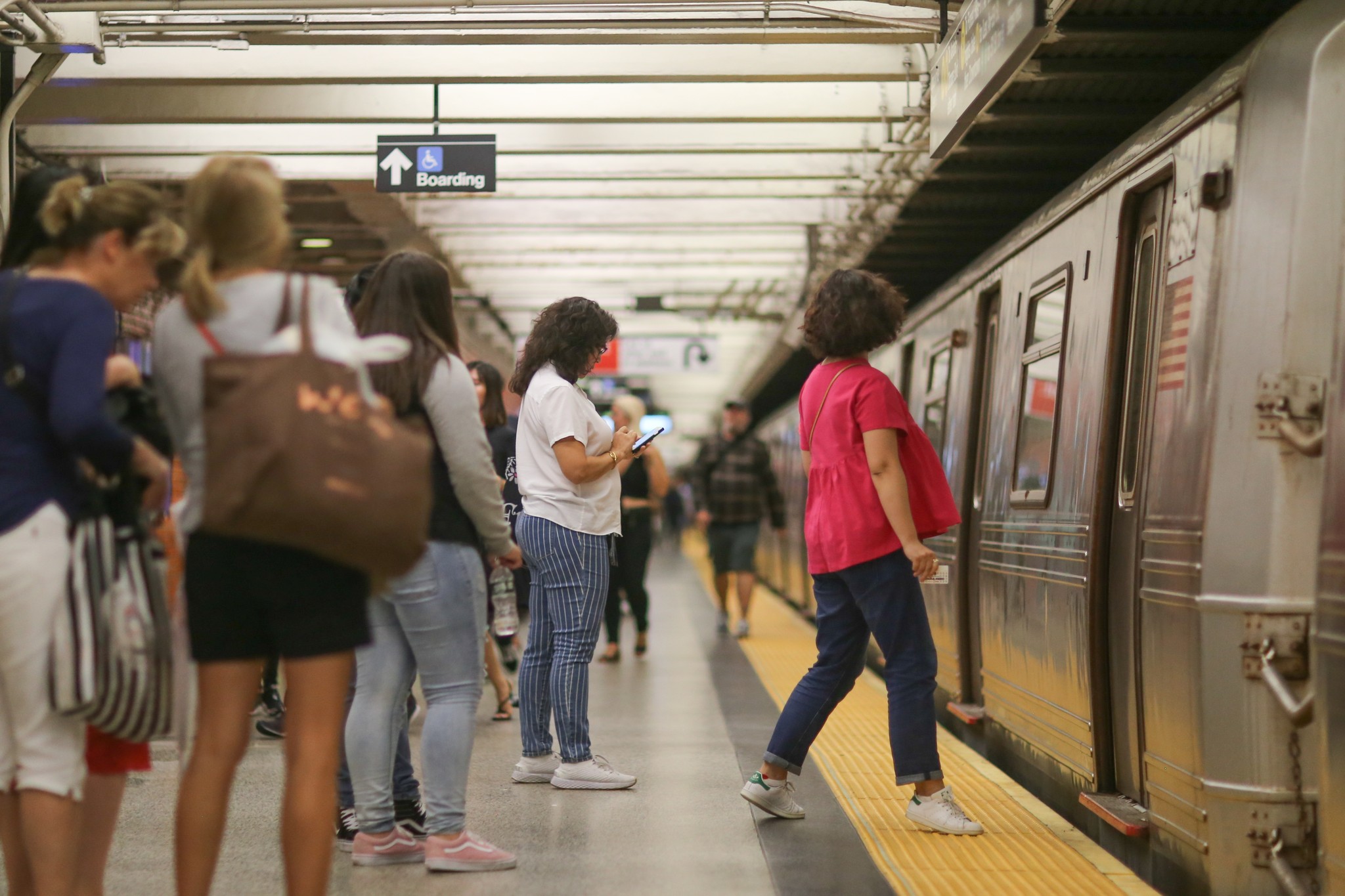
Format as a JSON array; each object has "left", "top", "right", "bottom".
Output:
[
  {"left": 334, "top": 807, "right": 359, "bottom": 852},
  {"left": 741, "top": 771, "right": 805, "bottom": 818},
  {"left": 512, "top": 750, "right": 561, "bottom": 783},
  {"left": 393, "top": 799, "right": 427, "bottom": 840},
  {"left": 551, "top": 755, "right": 637, "bottom": 789},
  {"left": 906, "top": 785, "right": 983, "bottom": 835},
  {"left": 352, "top": 824, "right": 425, "bottom": 865},
  {"left": 424, "top": 831, "right": 517, "bottom": 871}
]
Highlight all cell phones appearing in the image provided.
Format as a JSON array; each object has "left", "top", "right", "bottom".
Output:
[{"left": 630, "top": 428, "right": 664, "bottom": 454}]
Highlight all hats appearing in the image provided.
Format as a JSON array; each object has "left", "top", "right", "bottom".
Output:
[{"left": 724, "top": 396, "right": 750, "bottom": 411}]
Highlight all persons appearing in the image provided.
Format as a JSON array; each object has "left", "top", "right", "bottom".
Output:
[
  {"left": 1, "top": 180, "right": 188, "bottom": 896},
  {"left": 233, "top": 353, "right": 526, "bottom": 844},
  {"left": 346, "top": 248, "right": 526, "bottom": 876},
  {"left": 690, "top": 401, "right": 788, "bottom": 640},
  {"left": 503, "top": 295, "right": 637, "bottom": 792},
  {"left": 595, "top": 394, "right": 671, "bottom": 665},
  {"left": 146, "top": 150, "right": 390, "bottom": 896},
  {"left": 5, "top": 162, "right": 184, "bottom": 896},
  {"left": 739, "top": 265, "right": 984, "bottom": 838}
]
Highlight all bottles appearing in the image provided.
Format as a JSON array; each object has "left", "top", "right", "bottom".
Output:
[{"left": 490, "top": 559, "right": 519, "bottom": 636}]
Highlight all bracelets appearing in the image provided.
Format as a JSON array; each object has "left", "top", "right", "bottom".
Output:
[{"left": 606, "top": 450, "right": 618, "bottom": 470}]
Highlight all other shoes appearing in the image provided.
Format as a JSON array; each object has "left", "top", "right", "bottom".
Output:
[
  {"left": 251, "top": 675, "right": 287, "bottom": 737},
  {"left": 635, "top": 643, "right": 647, "bottom": 655},
  {"left": 600, "top": 651, "right": 621, "bottom": 663},
  {"left": 736, "top": 618, "right": 749, "bottom": 639},
  {"left": 714, "top": 608, "right": 729, "bottom": 634}
]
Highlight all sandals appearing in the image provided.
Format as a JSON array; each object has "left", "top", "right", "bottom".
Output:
[{"left": 491, "top": 680, "right": 514, "bottom": 721}]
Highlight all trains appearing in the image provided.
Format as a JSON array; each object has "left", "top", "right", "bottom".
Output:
[{"left": 761, "top": 0, "right": 1344, "bottom": 896}]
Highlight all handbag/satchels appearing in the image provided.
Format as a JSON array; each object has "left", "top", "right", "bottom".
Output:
[
  {"left": 199, "top": 270, "right": 432, "bottom": 581},
  {"left": 49, "top": 507, "right": 173, "bottom": 743}
]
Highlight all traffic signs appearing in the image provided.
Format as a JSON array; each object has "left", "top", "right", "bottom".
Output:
[{"left": 376, "top": 133, "right": 496, "bottom": 192}]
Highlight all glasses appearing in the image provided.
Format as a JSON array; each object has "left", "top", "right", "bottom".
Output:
[{"left": 600, "top": 345, "right": 608, "bottom": 354}]
